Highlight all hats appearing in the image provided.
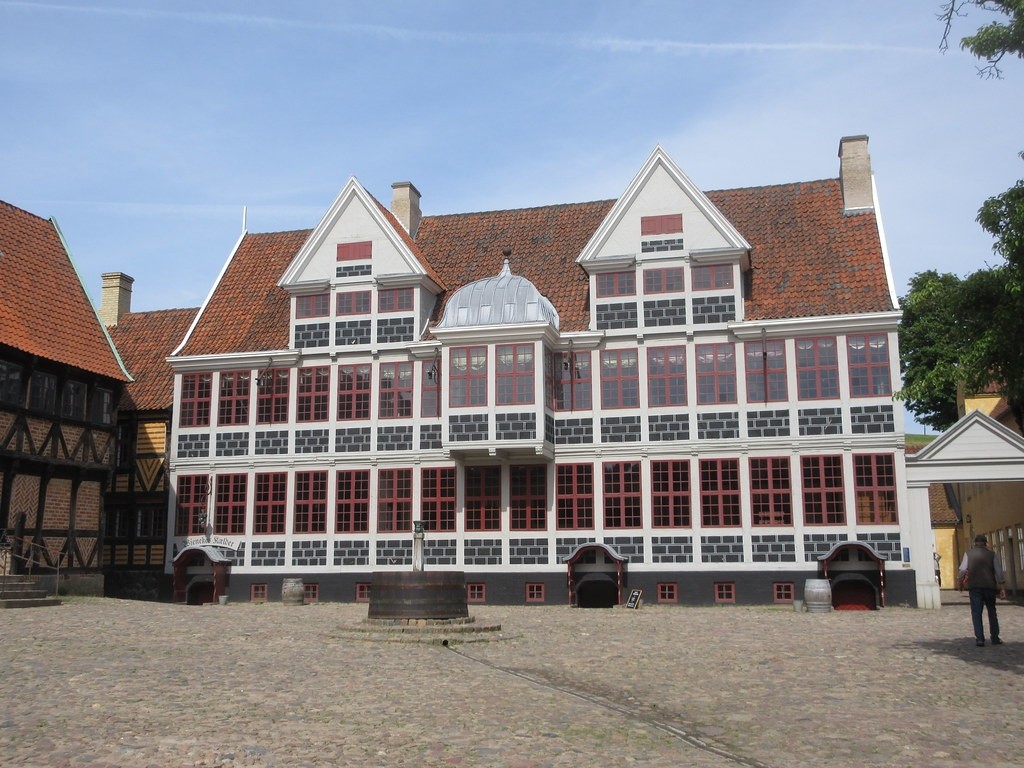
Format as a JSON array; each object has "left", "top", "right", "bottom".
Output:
[{"left": 975, "top": 535, "right": 987, "bottom": 542}]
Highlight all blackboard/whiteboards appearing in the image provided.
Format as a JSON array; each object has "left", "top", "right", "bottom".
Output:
[{"left": 626, "top": 589, "right": 642, "bottom": 606}]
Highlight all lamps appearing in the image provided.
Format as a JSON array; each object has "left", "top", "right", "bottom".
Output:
[
  {"left": 563, "top": 338, "right": 576, "bottom": 370},
  {"left": 425, "top": 348, "right": 438, "bottom": 381},
  {"left": 255, "top": 357, "right": 274, "bottom": 386}
]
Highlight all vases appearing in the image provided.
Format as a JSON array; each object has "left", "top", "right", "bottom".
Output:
[{"left": 219, "top": 595, "right": 229, "bottom": 605}]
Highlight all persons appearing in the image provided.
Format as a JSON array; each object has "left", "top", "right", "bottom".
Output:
[{"left": 958, "top": 534, "right": 1006, "bottom": 646}]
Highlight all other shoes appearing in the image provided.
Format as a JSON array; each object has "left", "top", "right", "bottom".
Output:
[
  {"left": 977, "top": 640, "right": 984, "bottom": 647},
  {"left": 991, "top": 638, "right": 1003, "bottom": 644}
]
